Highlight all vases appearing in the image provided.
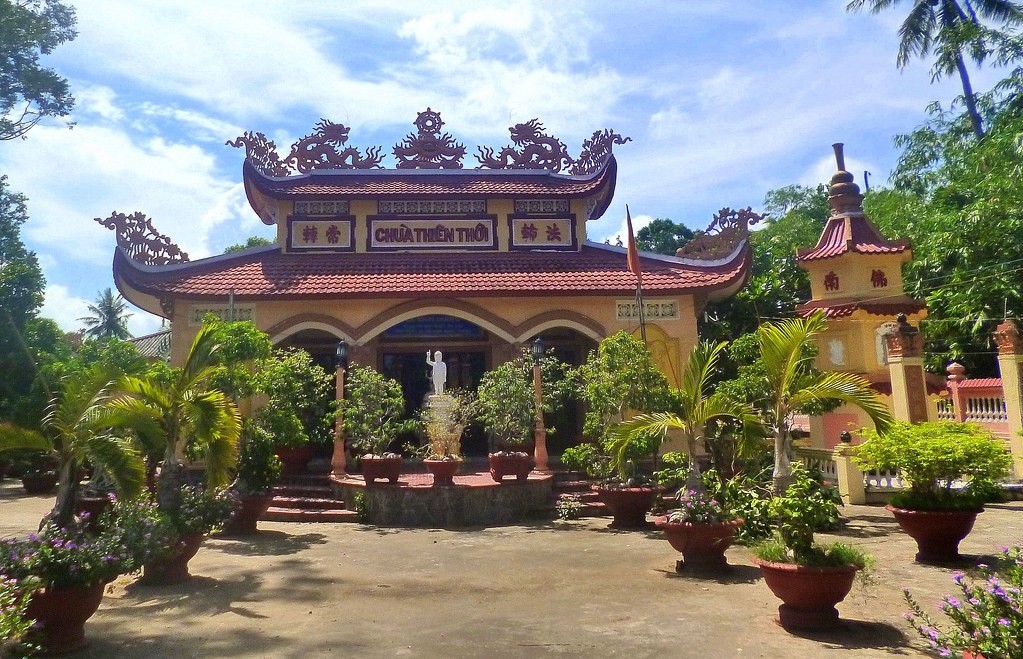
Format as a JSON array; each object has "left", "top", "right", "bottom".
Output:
[
  {"left": 72, "top": 497, "right": 110, "bottom": 534},
  {"left": 654, "top": 515, "right": 746, "bottom": 577},
  {"left": 18, "top": 577, "right": 117, "bottom": 654},
  {"left": 141, "top": 533, "right": 204, "bottom": 585}
]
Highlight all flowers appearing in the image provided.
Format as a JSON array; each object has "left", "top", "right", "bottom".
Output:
[
  {"left": 902, "top": 545, "right": 1023, "bottom": 659},
  {"left": 671, "top": 489, "right": 723, "bottom": 526},
  {"left": 0, "top": 460, "right": 244, "bottom": 659}
]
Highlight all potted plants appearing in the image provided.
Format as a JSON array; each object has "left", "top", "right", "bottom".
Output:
[
  {"left": 846, "top": 417, "right": 1016, "bottom": 563},
  {"left": 560, "top": 328, "right": 696, "bottom": 531},
  {"left": 470, "top": 347, "right": 575, "bottom": 481},
  {"left": 750, "top": 460, "right": 877, "bottom": 632},
  {"left": 329, "top": 366, "right": 419, "bottom": 484},
  {"left": 202, "top": 313, "right": 273, "bottom": 535},
  {"left": 266, "top": 345, "right": 333, "bottom": 473},
  {"left": 419, "top": 386, "right": 476, "bottom": 487}
]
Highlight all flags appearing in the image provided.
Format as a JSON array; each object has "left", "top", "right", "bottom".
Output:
[{"left": 627, "top": 212, "right": 642, "bottom": 284}]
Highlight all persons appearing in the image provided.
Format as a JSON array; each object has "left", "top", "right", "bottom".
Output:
[{"left": 425, "top": 349, "right": 447, "bottom": 394}]
